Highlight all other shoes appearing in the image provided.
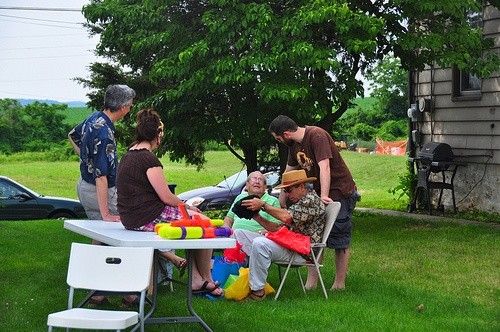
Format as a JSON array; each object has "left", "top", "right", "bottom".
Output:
[
  {"left": 88, "top": 297, "right": 115, "bottom": 309},
  {"left": 122, "top": 296, "right": 140, "bottom": 308},
  {"left": 242, "top": 291, "right": 266, "bottom": 302}
]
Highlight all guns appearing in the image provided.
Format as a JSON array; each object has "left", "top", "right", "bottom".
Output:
[{"left": 155, "top": 204, "right": 236, "bottom": 239}]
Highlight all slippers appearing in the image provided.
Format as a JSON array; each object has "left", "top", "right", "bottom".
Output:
[
  {"left": 178, "top": 259, "right": 188, "bottom": 278},
  {"left": 209, "top": 287, "right": 225, "bottom": 298},
  {"left": 192, "top": 281, "right": 221, "bottom": 294}
]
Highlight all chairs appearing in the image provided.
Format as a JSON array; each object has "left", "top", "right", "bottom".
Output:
[
  {"left": 271, "top": 200, "right": 342, "bottom": 301},
  {"left": 47, "top": 242, "right": 154, "bottom": 332}
]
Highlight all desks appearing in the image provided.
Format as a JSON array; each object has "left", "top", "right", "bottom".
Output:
[{"left": 63, "top": 214, "right": 239, "bottom": 332}]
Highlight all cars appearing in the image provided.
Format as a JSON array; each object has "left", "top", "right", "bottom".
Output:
[
  {"left": 0, "top": 176, "right": 88, "bottom": 220},
  {"left": 175, "top": 164, "right": 362, "bottom": 212}
]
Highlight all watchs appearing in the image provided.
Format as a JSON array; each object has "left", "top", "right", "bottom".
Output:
[{"left": 260, "top": 202, "right": 267, "bottom": 211}]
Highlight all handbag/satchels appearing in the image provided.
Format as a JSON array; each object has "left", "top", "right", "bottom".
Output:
[
  {"left": 211, "top": 256, "right": 277, "bottom": 301},
  {"left": 265, "top": 225, "right": 312, "bottom": 255}
]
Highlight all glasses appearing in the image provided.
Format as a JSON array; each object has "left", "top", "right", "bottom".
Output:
[
  {"left": 275, "top": 129, "right": 291, "bottom": 142},
  {"left": 249, "top": 178, "right": 265, "bottom": 184},
  {"left": 283, "top": 183, "right": 301, "bottom": 193}
]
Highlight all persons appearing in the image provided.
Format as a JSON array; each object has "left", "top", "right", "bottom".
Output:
[
  {"left": 151, "top": 247, "right": 188, "bottom": 296},
  {"left": 68, "top": 85, "right": 142, "bottom": 308},
  {"left": 231, "top": 170, "right": 326, "bottom": 303},
  {"left": 269, "top": 115, "right": 358, "bottom": 291},
  {"left": 221, "top": 171, "right": 282, "bottom": 236},
  {"left": 116, "top": 109, "right": 224, "bottom": 298}
]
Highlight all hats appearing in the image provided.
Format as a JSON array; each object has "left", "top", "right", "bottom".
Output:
[{"left": 275, "top": 169, "right": 317, "bottom": 189}]
